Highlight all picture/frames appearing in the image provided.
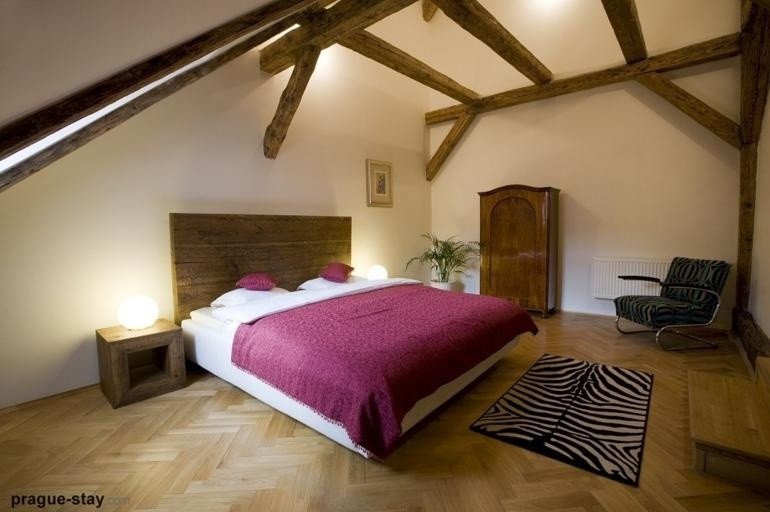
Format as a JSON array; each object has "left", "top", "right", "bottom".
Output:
[{"left": 366, "top": 159, "right": 393, "bottom": 208}]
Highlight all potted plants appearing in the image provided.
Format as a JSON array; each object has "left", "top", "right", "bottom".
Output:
[{"left": 404, "top": 230, "right": 480, "bottom": 291}]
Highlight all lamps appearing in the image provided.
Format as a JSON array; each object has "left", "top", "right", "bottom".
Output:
[{"left": 116, "top": 294, "right": 160, "bottom": 330}]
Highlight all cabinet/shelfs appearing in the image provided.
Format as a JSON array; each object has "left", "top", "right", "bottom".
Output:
[{"left": 476, "top": 184, "right": 561, "bottom": 319}]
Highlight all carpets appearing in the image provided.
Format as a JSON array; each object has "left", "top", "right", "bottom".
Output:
[{"left": 470, "top": 352, "right": 654, "bottom": 489}]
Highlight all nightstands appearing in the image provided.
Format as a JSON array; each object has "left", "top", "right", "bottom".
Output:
[{"left": 95, "top": 318, "right": 187, "bottom": 410}]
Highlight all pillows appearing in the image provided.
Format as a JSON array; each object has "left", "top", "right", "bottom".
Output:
[
  {"left": 319, "top": 262, "right": 354, "bottom": 283},
  {"left": 234, "top": 272, "right": 276, "bottom": 291},
  {"left": 209, "top": 286, "right": 290, "bottom": 308},
  {"left": 297, "top": 275, "right": 368, "bottom": 290}
]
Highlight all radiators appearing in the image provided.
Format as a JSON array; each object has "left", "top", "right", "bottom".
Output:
[{"left": 590, "top": 257, "right": 673, "bottom": 301}]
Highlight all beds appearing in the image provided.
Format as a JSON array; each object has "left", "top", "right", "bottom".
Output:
[{"left": 169, "top": 211, "right": 539, "bottom": 460}]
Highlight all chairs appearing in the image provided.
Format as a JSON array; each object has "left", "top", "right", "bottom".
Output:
[{"left": 613, "top": 256, "right": 734, "bottom": 352}]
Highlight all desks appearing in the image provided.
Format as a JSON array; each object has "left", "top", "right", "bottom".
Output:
[{"left": 688, "top": 369, "right": 770, "bottom": 472}]
